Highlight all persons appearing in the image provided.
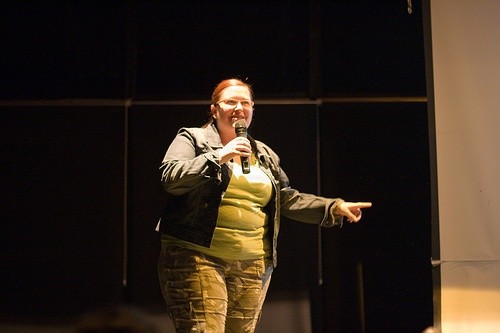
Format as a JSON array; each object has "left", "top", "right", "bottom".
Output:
[{"left": 156, "top": 77, "right": 372, "bottom": 333}]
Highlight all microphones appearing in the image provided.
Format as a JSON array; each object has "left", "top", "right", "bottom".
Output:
[{"left": 235, "top": 120, "right": 251, "bottom": 174}]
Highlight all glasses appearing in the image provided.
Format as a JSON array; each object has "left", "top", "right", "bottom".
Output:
[{"left": 216, "top": 98, "right": 254, "bottom": 108}]
